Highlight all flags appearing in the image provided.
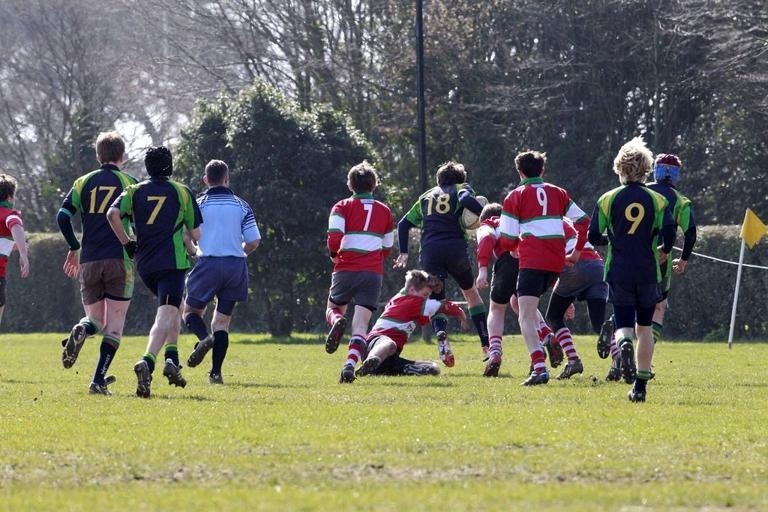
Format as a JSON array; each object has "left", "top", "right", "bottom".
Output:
[{"left": 739, "top": 208, "right": 768, "bottom": 250}]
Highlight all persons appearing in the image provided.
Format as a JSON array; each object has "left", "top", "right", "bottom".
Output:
[
  {"left": 356, "top": 269, "right": 468, "bottom": 379},
  {"left": 324, "top": 161, "right": 394, "bottom": 384},
  {"left": 107, "top": 146, "right": 204, "bottom": 398},
  {"left": 588, "top": 136, "right": 677, "bottom": 402},
  {"left": 475, "top": 202, "right": 575, "bottom": 377},
  {"left": 500, "top": 151, "right": 591, "bottom": 385},
  {"left": 596, "top": 152, "right": 697, "bottom": 379},
  {"left": 183, "top": 160, "right": 262, "bottom": 384},
  {"left": 392, "top": 162, "right": 491, "bottom": 366},
  {"left": 546, "top": 215, "right": 614, "bottom": 380},
  {"left": 58, "top": 131, "right": 138, "bottom": 395},
  {"left": 0, "top": 173, "right": 30, "bottom": 322}
]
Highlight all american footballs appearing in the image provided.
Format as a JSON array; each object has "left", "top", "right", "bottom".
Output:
[{"left": 460, "top": 194, "right": 489, "bottom": 230}]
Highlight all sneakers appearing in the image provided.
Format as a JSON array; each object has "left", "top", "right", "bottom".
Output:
[
  {"left": 208, "top": 369, "right": 223, "bottom": 384},
  {"left": 339, "top": 365, "right": 356, "bottom": 384},
  {"left": 529, "top": 347, "right": 547, "bottom": 375},
  {"left": 627, "top": 387, "right": 646, "bottom": 403},
  {"left": 555, "top": 359, "right": 583, "bottom": 381},
  {"left": 543, "top": 333, "right": 564, "bottom": 368},
  {"left": 482, "top": 354, "right": 502, "bottom": 377},
  {"left": 355, "top": 356, "right": 381, "bottom": 377},
  {"left": 518, "top": 371, "right": 549, "bottom": 386},
  {"left": 88, "top": 375, "right": 116, "bottom": 396},
  {"left": 163, "top": 358, "right": 187, "bottom": 388},
  {"left": 482, "top": 345, "right": 491, "bottom": 362},
  {"left": 606, "top": 354, "right": 623, "bottom": 382},
  {"left": 619, "top": 339, "right": 637, "bottom": 384},
  {"left": 60, "top": 323, "right": 87, "bottom": 369},
  {"left": 650, "top": 369, "right": 656, "bottom": 380},
  {"left": 403, "top": 364, "right": 441, "bottom": 376},
  {"left": 325, "top": 317, "right": 347, "bottom": 354},
  {"left": 187, "top": 334, "right": 215, "bottom": 368},
  {"left": 596, "top": 320, "right": 614, "bottom": 360},
  {"left": 133, "top": 360, "right": 153, "bottom": 399},
  {"left": 436, "top": 331, "right": 455, "bottom": 368}
]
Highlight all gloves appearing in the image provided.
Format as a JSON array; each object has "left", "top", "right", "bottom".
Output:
[{"left": 123, "top": 239, "right": 137, "bottom": 260}]
